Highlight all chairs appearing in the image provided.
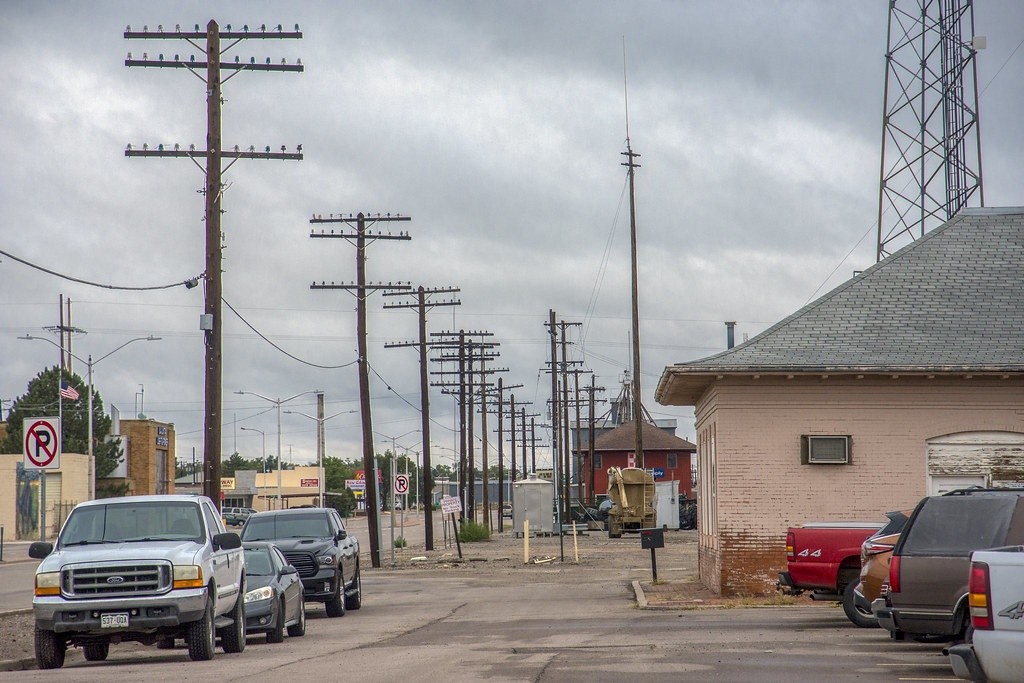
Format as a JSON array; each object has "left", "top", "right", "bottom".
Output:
[{"left": 167, "top": 519, "right": 197, "bottom": 535}]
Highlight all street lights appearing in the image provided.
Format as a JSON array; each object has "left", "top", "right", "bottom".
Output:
[
  {"left": 283, "top": 407, "right": 359, "bottom": 508},
  {"left": 231, "top": 389, "right": 327, "bottom": 508},
  {"left": 239, "top": 425, "right": 266, "bottom": 511},
  {"left": 16, "top": 332, "right": 163, "bottom": 498},
  {"left": 372, "top": 427, "right": 483, "bottom": 560}
]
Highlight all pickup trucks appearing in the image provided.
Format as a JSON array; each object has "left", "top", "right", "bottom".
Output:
[
  {"left": 870, "top": 480, "right": 1024, "bottom": 647},
  {"left": 948, "top": 544, "right": 1024, "bottom": 683},
  {"left": 776, "top": 523, "right": 891, "bottom": 629}
]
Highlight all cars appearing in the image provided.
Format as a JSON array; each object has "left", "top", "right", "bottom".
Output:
[
  {"left": 221, "top": 506, "right": 258, "bottom": 527},
  {"left": 240, "top": 540, "right": 307, "bottom": 644},
  {"left": 850, "top": 507, "right": 914, "bottom": 609}
]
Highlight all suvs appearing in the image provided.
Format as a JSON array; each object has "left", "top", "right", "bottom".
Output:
[
  {"left": 239, "top": 505, "right": 363, "bottom": 619},
  {"left": 27, "top": 495, "right": 246, "bottom": 664}
]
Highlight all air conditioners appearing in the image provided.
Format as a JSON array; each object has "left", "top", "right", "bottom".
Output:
[{"left": 808, "top": 436, "right": 849, "bottom": 464}]
